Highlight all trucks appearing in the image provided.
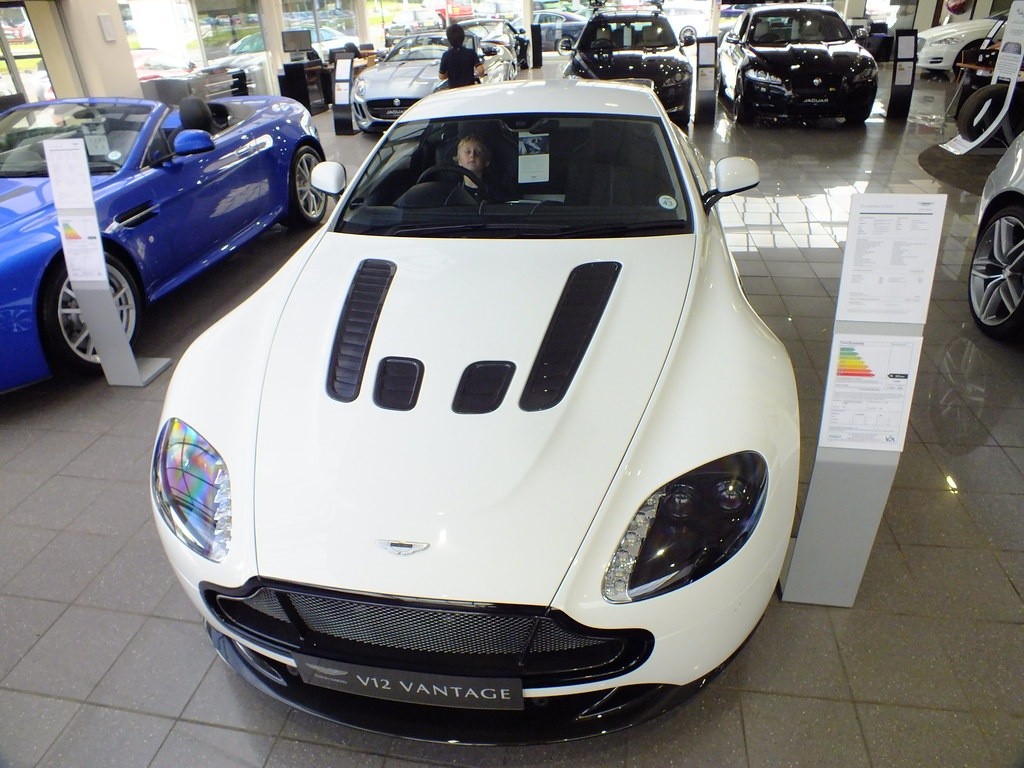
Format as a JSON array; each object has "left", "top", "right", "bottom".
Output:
[{"left": 436, "top": 0, "right": 511, "bottom": 27}]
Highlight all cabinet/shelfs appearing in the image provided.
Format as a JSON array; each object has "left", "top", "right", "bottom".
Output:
[{"left": 154, "top": 66, "right": 248, "bottom": 98}]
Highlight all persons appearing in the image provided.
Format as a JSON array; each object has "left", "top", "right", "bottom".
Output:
[
  {"left": 439, "top": 24, "right": 484, "bottom": 89},
  {"left": 458, "top": 133, "right": 506, "bottom": 204}
]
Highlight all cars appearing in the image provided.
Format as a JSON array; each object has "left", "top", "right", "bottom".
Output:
[
  {"left": 720, "top": 4, "right": 764, "bottom": 18},
  {"left": 215, "top": 25, "right": 357, "bottom": 71},
  {"left": 562, "top": 8, "right": 695, "bottom": 122},
  {"left": 662, "top": 6, "right": 709, "bottom": 45},
  {"left": 718, "top": 7, "right": 878, "bottom": 129},
  {"left": 966, "top": 126, "right": 1024, "bottom": 337},
  {"left": 512, "top": 10, "right": 589, "bottom": 55},
  {"left": 386, "top": 7, "right": 444, "bottom": 45},
  {"left": 916, "top": 6, "right": 1014, "bottom": 75}
]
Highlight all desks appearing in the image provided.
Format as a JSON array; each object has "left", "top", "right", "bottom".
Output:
[
  {"left": 942, "top": 62, "right": 996, "bottom": 125},
  {"left": 323, "top": 61, "right": 368, "bottom": 68}
]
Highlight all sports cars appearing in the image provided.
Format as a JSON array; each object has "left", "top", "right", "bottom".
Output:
[
  {"left": 0, "top": 97, "right": 331, "bottom": 395},
  {"left": 148, "top": 72, "right": 803, "bottom": 747},
  {"left": 350, "top": 28, "right": 518, "bottom": 136}
]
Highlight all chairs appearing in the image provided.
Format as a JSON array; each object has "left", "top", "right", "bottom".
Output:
[
  {"left": 329, "top": 47, "right": 348, "bottom": 64},
  {"left": 345, "top": 43, "right": 360, "bottom": 59},
  {"left": 595, "top": 25, "right": 665, "bottom": 51},
  {"left": 167, "top": 98, "right": 222, "bottom": 151},
  {"left": 436, "top": 136, "right": 522, "bottom": 200},
  {"left": 754, "top": 19, "right": 826, "bottom": 42},
  {"left": 360, "top": 44, "right": 374, "bottom": 50},
  {"left": 564, "top": 121, "right": 657, "bottom": 205}
]
